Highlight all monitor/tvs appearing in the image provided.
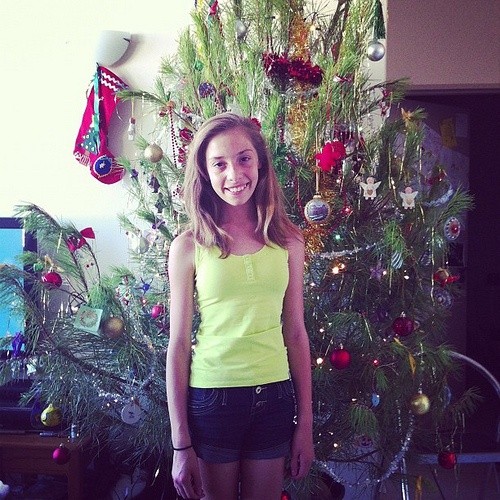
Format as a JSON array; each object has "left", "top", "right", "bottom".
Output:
[{"left": 0, "top": 217, "right": 38, "bottom": 360}]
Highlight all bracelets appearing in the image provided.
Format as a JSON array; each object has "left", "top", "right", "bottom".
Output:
[{"left": 173, "top": 445, "right": 193, "bottom": 451}]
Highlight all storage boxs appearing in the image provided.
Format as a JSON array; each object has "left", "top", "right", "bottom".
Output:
[{"left": 0, "top": 379, "right": 67, "bottom": 431}]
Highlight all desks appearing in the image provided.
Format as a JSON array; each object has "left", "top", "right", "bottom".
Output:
[{"left": 0, "top": 421, "right": 109, "bottom": 500}]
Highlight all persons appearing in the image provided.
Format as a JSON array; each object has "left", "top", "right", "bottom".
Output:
[{"left": 166, "top": 113, "right": 315, "bottom": 500}]
